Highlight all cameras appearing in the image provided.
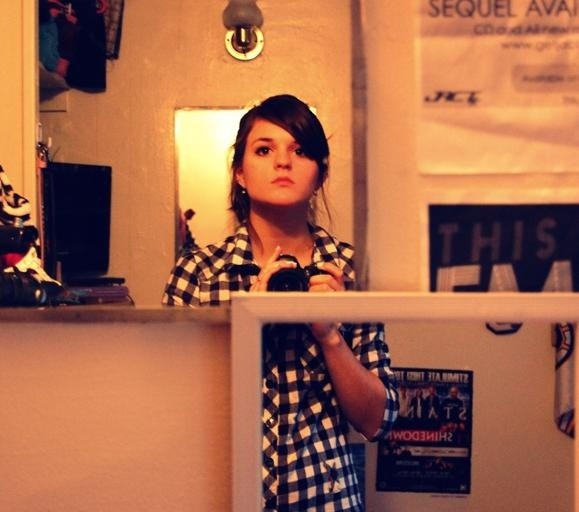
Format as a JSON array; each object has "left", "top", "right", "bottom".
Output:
[{"left": 267, "top": 265, "right": 319, "bottom": 292}]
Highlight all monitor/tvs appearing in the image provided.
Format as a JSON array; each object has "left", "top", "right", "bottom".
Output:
[{"left": 36, "top": 161, "right": 127, "bottom": 284}]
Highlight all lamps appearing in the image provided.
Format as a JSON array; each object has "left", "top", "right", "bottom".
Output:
[{"left": 221, "top": 0, "right": 265, "bottom": 62}]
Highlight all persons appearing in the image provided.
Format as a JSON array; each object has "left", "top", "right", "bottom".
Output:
[
  {"left": 161, "top": 95, "right": 400, "bottom": 512},
  {"left": 399, "top": 385, "right": 464, "bottom": 422}
]
